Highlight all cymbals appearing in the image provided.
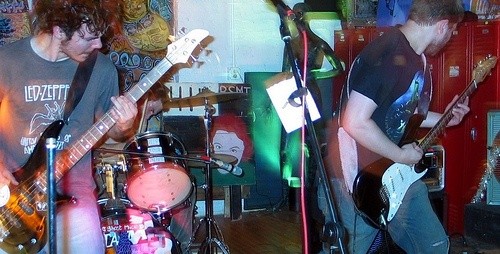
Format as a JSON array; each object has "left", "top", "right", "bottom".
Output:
[{"left": 162, "top": 88, "right": 248, "bottom": 109}]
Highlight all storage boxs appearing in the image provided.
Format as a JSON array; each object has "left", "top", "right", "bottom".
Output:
[{"left": 462, "top": 204, "right": 500, "bottom": 254}]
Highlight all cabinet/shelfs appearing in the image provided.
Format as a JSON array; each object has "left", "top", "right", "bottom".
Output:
[{"left": 333, "top": 18, "right": 500, "bottom": 236}]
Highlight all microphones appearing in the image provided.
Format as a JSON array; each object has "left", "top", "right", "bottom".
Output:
[
  {"left": 211, "top": 158, "right": 245, "bottom": 178},
  {"left": 271, "top": 0, "right": 296, "bottom": 20}
]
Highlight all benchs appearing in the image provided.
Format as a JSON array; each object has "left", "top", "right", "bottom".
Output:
[{"left": 162, "top": 117, "right": 256, "bottom": 221}]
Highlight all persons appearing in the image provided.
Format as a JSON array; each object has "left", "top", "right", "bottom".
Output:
[
  {"left": 93, "top": 83, "right": 169, "bottom": 165},
  {"left": 317, "top": 0, "right": 470, "bottom": 254},
  {"left": 0, "top": 0, "right": 138, "bottom": 254}
]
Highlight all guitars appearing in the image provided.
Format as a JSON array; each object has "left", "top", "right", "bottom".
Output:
[
  {"left": 0, "top": 27, "right": 210, "bottom": 253},
  {"left": 353, "top": 54, "right": 497, "bottom": 230}
]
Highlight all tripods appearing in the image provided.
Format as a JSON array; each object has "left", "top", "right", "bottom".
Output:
[{"left": 193, "top": 97, "right": 230, "bottom": 254}]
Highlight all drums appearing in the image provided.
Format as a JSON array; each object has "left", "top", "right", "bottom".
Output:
[
  {"left": 97, "top": 199, "right": 177, "bottom": 254},
  {"left": 124, "top": 131, "right": 193, "bottom": 214}
]
